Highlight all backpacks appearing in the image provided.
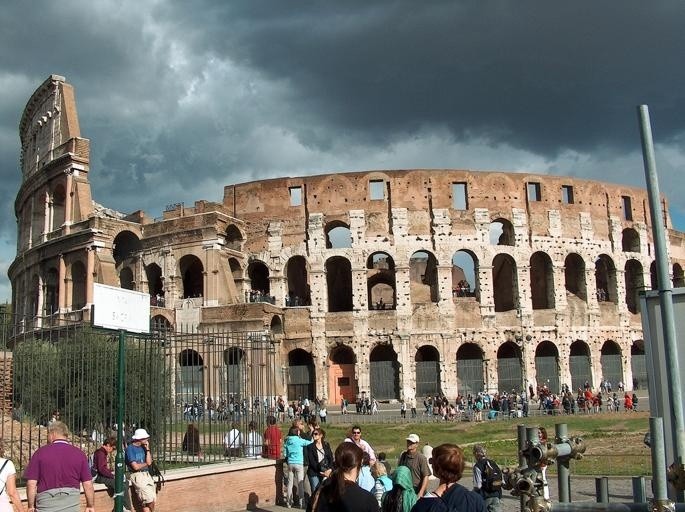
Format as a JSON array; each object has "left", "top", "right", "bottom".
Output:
[{"left": 481, "top": 457, "right": 502, "bottom": 492}]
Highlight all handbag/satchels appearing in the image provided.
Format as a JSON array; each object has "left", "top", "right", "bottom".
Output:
[{"left": 321, "top": 458, "right": 329, "bottom": 471}]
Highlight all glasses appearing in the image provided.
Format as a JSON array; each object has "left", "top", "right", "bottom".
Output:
[
  {"left": 352, "top": 431, "right": 361, "bottom": 435},
  {"left": 312, "top": 432, "right": 319, "bottom": 435}
]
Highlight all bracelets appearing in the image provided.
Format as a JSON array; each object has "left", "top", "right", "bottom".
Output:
[{"left": 145, "top": 449, "right": 150, "bottom": 451}]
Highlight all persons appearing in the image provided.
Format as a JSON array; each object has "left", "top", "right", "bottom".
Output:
[
  {"left": 539, "top": 427, "right": 550, "bottom": 499},
  {"left": 22, "top": 421, "right": 94, "bottom": 512},
  {"left": 454, "top": 279, "right": 472, "bottom": 296},
  {"left": 597, "top": 288, "right": 607, "bottom": 301},
  {"left": 288, "top": 393, "right": 329, "bottom": 423},
  {"left": 529, "top": 378, "right": 639, "bottom": 415},
  {"left": 148, "top": 287, "right": 310, "bottom": 309},
  {"left": 422, "top": 389, "right": 528, "bottom": 422},
  {"left": 182, "top": 393, "right": 285, "bottom": 460},
  {"left": 401, "top": 398, "right": 417, "bottom": 418},
  {"left": 47, "top": 408, "right": 137, "bottom": 447},
  {"left": 125, "top": 429, "right": 157, "bottom": 512},
  {"left": 306, "top": 425, "right": 503, "bottom": 512},
  {"left": 282, "top": 420, "right": 333, "bottom": 508},
  {"left": 0, "top": 457, "right": 27, "bottom": 512},
  {"left": 91, "top": 437, "right": 136, "bottom": 512},
  {"left": 341, "top": 396, "right": 378, "bottom": 415}
]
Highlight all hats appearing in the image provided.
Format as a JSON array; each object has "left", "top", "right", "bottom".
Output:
[
  {"left": 406, "top": 434, "right": 420, "bottom": 444},
  {"left": 132, "top": 428, "right": 150, "bottom": 440}
]
